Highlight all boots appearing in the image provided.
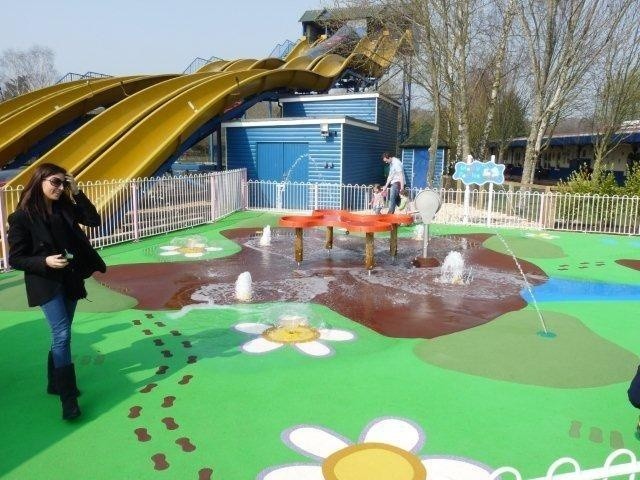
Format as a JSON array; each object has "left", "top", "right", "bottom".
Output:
[{"left": 46, "top": 350, "right": 81, "bottom": 420}]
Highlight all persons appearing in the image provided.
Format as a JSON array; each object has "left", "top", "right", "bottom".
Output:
[
  {"left": 396, "top": 188, "right": 412, "bottom": 217},
  {"left": 367, "top": 184, "right": 387, "bottom": 214},
  {"left": 380, "top": 151, "right": 407, "bottom": 215},
  {"left": 6, "top": 160, "right": 108, "bottom": 422}
]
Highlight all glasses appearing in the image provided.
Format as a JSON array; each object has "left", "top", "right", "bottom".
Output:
[{"left": 41, "top": 176, "right": 70, "bottom": 187}]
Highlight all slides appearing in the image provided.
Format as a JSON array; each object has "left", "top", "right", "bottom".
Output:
[
  {"left": 66, "top": 28, "right": 418, "bottom": 230},
  {"left": 1, "top": 32, "right": 390, "bottom": 237}
]
